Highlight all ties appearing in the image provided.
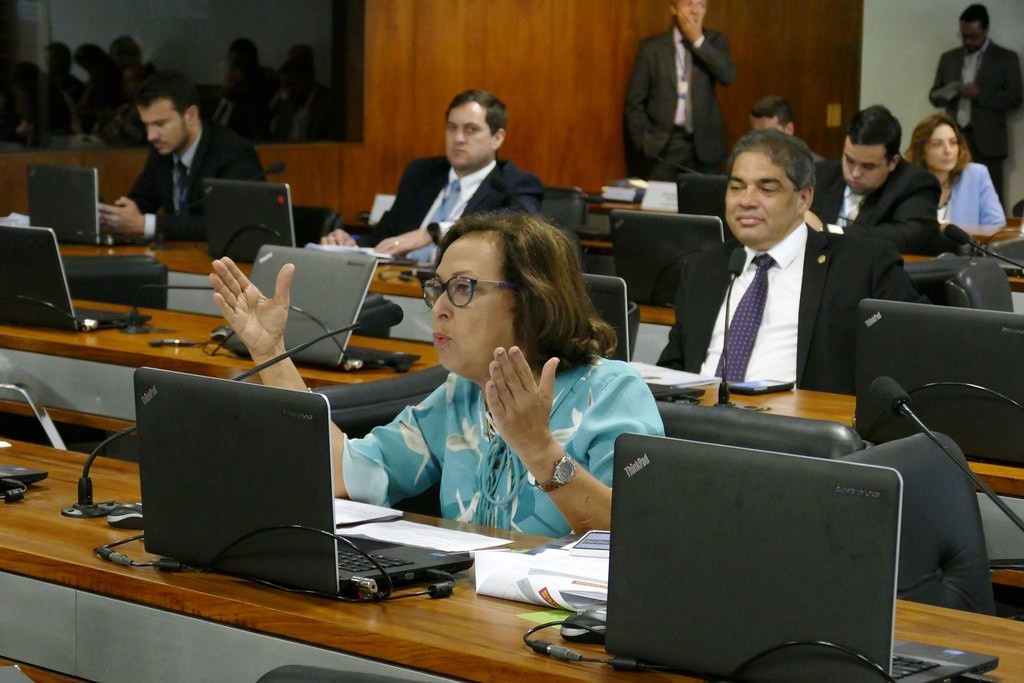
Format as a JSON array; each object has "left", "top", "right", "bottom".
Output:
[
  {"left": 176, "top": 162, "right": 189, "bottom": 211},
  {"left": 714, "top": 253, "right": 775, "bottom": 383},
  {"left": 955, "top": 53, "right": 977, "bottom": 129},
  {"left": 409, "top": 179, "right": 461, "bottom": 263},
  {"left": 682, "top": 44, "right": 695, "bottom": 134}
]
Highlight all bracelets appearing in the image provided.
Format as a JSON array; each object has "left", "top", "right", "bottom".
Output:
[{"left": 395, "top": 242, "right": 399, "bottom": 246}]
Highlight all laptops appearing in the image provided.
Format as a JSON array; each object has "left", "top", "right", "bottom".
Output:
[
  {"left": 0, "top": 162, "right": 473, "bottom": 599},
  {"left": 576, "top": 172, "right": 1024, "bottom": 683}
]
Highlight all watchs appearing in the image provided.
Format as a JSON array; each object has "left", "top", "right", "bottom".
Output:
[
  {"left": 534, "top": 452, "right": 576, "bottom": 493},
  {"left": 428, "top": 223, "right": 440, "bottom": 243}
]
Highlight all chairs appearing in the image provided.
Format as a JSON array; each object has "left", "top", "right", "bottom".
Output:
[
  {"left": 677, "top": 173, "right": 744, "bottom": 250},
  {"left": 653, "top": 397, "right": 864, "bottom": 459},
  {"left": 834, "top": 430, "right": 997, "bottom": 618},
  {"left": 904, "top": 258, "right": 1015, "bottom": 313},
  {"left": 0, "top": 382, "right": 69, "bottom": 451},
  {"left": 312, "top": 364, "right": 450, "bottom": 439},
  {"left": 293, "top": 206, "right": 346, "bottom": 248},
  {"left": 352, "top": 293, "right": 390, "bottom": 339},
  {"left": 59, "top": 254, "right": 170, "bottom": 312},
  {"left": 582, "top": 272, "right": 639, "bottom": 364},
  {"left": 541, "top": 185, "right": 592, "bottom": 233}
]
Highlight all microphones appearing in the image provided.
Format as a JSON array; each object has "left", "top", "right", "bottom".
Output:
[
  {"left": 78, "top": 303, "right": 403, "bottom": 503},
  {"left": 944, "top": 224, "right": 1024, "bottom": 270},
  {"left": 870, "top": 375, "right": 1024, "bottom": 532},
  {"left": 544, "top": 193, "right": 606, "bottom": 203}
]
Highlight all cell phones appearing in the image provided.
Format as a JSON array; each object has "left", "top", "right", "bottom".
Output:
[
  {"left": 729, "top": 380, "right": 794, "bottom": 396},
  {"left": 98, "top": 195, "right": 106, "bottom": 203},
  {"left": 712, "top": 247, "right": 760, "bottom": 411}
]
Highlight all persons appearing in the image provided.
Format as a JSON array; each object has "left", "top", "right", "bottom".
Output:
[
  {"left": 804, "top": 105, "right": 942, "bottom": 251},
  {"left": 197, "top": 38, "right": 333, "bottom": 140},
  {"left": 656, "top": 130, "right": 914, "bottom": 394},
  {"left": 209, "top": 213, "right": 666, "bottom": 537},
  {"left": 929, "top": 4, "right": 1024, "bottom": 213},
  {"left": 321, "top": 90, "right": 543, "bottom": 263},
  {"left": 15, "top": 33, "right": 155, "bottom": 145},
  {"left": 624, "top": 0, "right": 734, "bottom": 181},
  {"left": 100, "top": 71, "right": 266, "bottom": 243},
  {"left": 748, "top": 94, "right": 825, "bottom": 164},
  {"left": 904, "top": 114, "right": 1007, "bottom": 227}
]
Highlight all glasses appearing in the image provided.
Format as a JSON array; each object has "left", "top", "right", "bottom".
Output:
[{"left": 421, "top": 276, "right": 519, "bottom": 308}]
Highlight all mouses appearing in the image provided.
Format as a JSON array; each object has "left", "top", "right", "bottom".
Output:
[
  {"left": 560, "top": 610, "right": 607, "bottom": 645},
  {"left": 107, "top": 502, "right": 145, "bottom": 531},
  {"left": 211, "top": 326, "right": 233, "bottom": 340}
]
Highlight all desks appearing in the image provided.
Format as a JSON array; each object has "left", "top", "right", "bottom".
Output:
[{"left": 0, "top": 202, "right": 1024, "bottom": 683}]
